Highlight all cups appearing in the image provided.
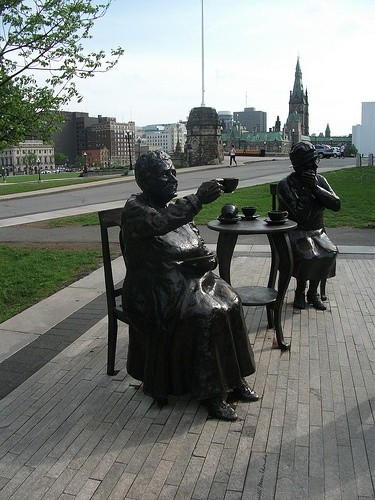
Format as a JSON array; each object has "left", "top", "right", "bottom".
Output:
[
  {"left": 216, "top": 177, "right": 239, "bottom": 193},
  {"left": 268, "top": 210, "right": 288, "bottom": 221},
  {"left": 242, "top": 207, "right": 257, "bottom": 217}
]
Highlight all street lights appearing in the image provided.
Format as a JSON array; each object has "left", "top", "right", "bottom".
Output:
[
  {"left": 124, "top": 130, "right": 134, "bottom": 170},
  {"left": 1, "top": 167, "right": 5, "bottom": 181},
  {"left": 36, "top": 158, "right": 41, "bottom": 180},
  {"left": 136, "top": 136, "right": 142, "bottom": 158},
  {"left": 83, "top": 152, "right": 87, "bottom": 172}
]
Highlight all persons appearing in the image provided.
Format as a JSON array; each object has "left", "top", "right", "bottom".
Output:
[
  {"left": 339, "top": 143, "right": 345, "bottom": 159},
  {"left": 277, "top": 141, "right": 340, "bottom": 310},
  {"left": 229, "top": 144, "right": 238, "bottom": 168},
  {"left": 120, "top": 150, "right": 259, "bottom": 419}
]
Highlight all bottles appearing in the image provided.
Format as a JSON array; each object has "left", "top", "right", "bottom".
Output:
[{"left": 221, "top": 203, "right": 238, "bottom": 219}]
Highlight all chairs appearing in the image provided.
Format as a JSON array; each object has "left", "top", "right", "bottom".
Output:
[
  {"left": 98, "top": 207, "right": 130, "bottom": 376},
  {"left": 270, "top": 183, "right": 328, "bottom": 301}
]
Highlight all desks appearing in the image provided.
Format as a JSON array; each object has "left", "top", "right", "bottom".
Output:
[{"left": 209, "top": 218, "right": 299, "bottom": 351}]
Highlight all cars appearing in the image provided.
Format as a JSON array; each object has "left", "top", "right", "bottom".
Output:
[
  {"left": 331, "top": 147, "right": 341, "bottom": 158},
  {"left": 40, "top": 168, "right": 71, "bottom": 174}
]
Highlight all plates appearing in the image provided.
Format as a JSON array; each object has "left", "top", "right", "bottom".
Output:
[
  {"left": 217, "top": 216, "right": 240, "bottom": 224},
  {"left": 241, "top": 216, "right": 258, "bottom": 220},
  {"left": 264, "top": 219, "right": 289, "bottom": 224}
]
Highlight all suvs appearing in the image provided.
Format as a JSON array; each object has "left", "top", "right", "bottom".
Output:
[{"left": 314, "top": 145, "right": 332, "bottom": 159}]
